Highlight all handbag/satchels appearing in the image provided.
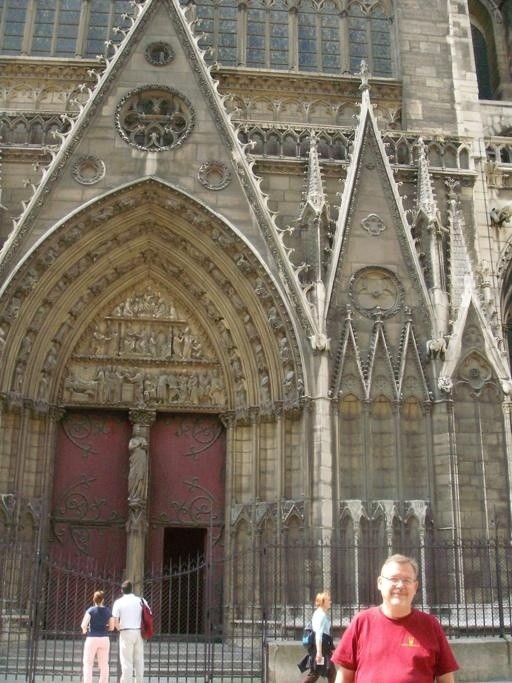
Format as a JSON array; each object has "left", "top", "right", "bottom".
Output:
[{"left": 142, "top": 605, "right": 154, "bottom": 638}]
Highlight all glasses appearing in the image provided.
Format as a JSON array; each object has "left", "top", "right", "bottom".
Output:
[{"left": 382, "top": 575, "right": 415, "bottom": 583}]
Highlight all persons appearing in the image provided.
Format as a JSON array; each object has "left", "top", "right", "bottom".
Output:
[
  {"left": 329, "top": 554, "right": 461, "bottom": 682},
  {"left": 303, "top": 588, "right": 337, "bottom": 682},
  {"left": 125, "top": 422, "right": 150, "bottom": 498},
  {"left": 111, "top": 579, "right": 152, "bottom": 683},
  {"left": 80, "top": 590, "right": 114, "bottom": 682}
]
